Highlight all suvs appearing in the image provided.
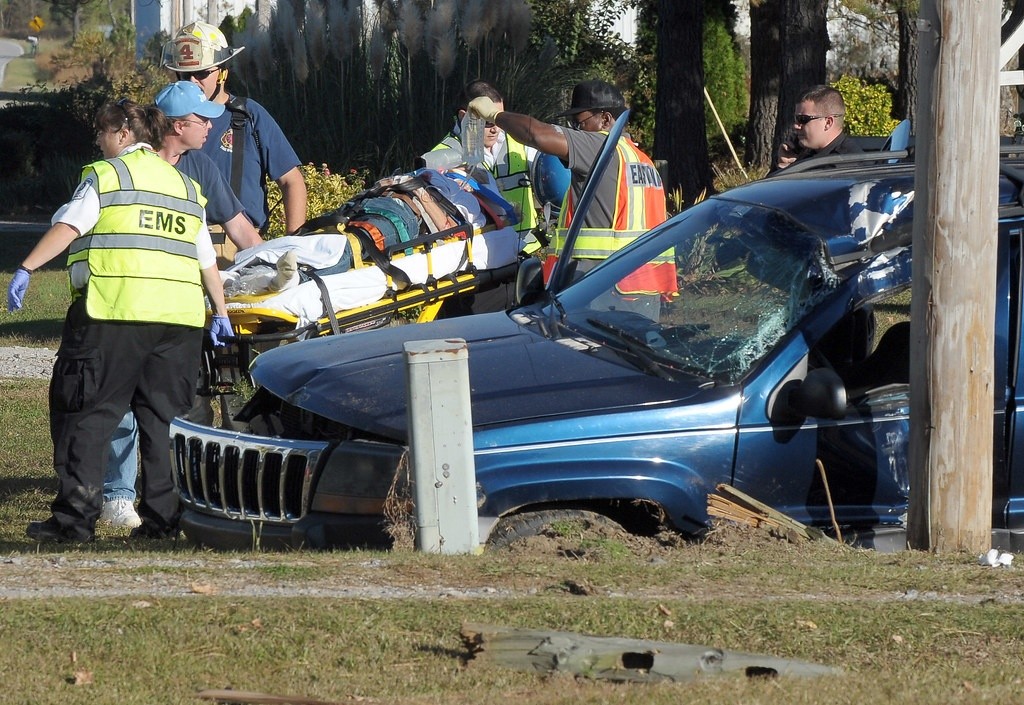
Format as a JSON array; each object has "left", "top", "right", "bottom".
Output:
[{"left": 170, "top": 141, "right": 1024, "bottom": 553}]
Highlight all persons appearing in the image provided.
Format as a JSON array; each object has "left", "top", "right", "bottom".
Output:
[
  {"left": 219, "top": 165, "right": 524, "bottom": 298},
  {"left": 9, "top": 95, "right": 230, "bottom": 544},
  {"left": 162, "top": 21, "right": 305, "bottom": 272},
  {"left": 469, "top": 79, "right": 678, "bottom": 325},
  {"left": 98, "top": 81, "right": 265, "bottom": 533},
  {"left": 767, "top": 86, "right": 876, "bottom": 363},
  {"left": 421, "top": 81, "right": 548, "bottom": 316}
]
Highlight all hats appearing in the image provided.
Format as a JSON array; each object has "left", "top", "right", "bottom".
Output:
[
  {"left": 154, "top": 80, "right": 225, "bottom": 119},
  {"left": 560, "top": 80, "right": 624, "bottom": 117}
]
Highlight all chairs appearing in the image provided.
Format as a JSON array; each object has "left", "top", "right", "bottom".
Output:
[{"left": 844, "top": 320, "right": 911, "bottom": 400}]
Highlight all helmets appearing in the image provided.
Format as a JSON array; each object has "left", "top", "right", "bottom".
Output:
[{"left": 160, "top": 21, "right": 245, "bottom": 72}]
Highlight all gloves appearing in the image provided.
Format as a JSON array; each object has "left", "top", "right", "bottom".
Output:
[
  {"left": 7, "top": 268, "right": 30, "bottom": 312},
  {"left": 467, "top": 96, "right": 504, "bottom": 125},
  {"left": 210, "top": 315, "right": 233, "bottom": 348}
]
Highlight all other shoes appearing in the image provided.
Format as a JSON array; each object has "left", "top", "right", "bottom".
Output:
[
  {"left": 129, "top": 526, "right": 177, "bottom": 548},
  {"left": 26, "top": 518, "right": 94, "bottom": 544}
]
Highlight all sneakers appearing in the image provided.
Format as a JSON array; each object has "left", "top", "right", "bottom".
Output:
[{"left": 100, "top": 499, "right": 141, "bottom": 529}]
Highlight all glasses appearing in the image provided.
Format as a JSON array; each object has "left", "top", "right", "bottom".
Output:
[
  {"left": 115, "top": 97, "right": 132, "bottom": 128},
  {"left": 572, "top": 111, "right": 605, "bottom": 131},
  {"left": 176, "top": 68, "right": 220, "bottom": 81},
  {"left": 793, "top": 114, "right": 838, "bottom": 124}
]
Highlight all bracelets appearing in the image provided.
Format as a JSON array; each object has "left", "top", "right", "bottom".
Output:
[{"left": 15, "top": 261, "right": 33, "bottom": 274}]
